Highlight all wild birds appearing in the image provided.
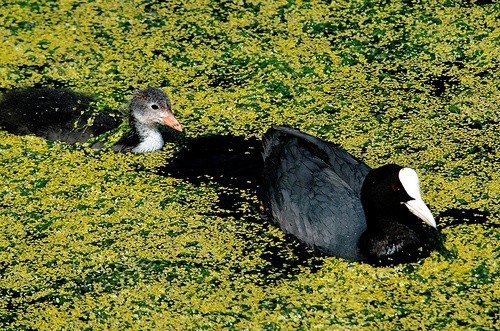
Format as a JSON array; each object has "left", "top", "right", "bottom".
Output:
[
  {"left": 258, "top": 124, "right": 436, "bottom": 259},
  {"left": 0, "top": 83, "right": 183, "bottom": 154}
]
